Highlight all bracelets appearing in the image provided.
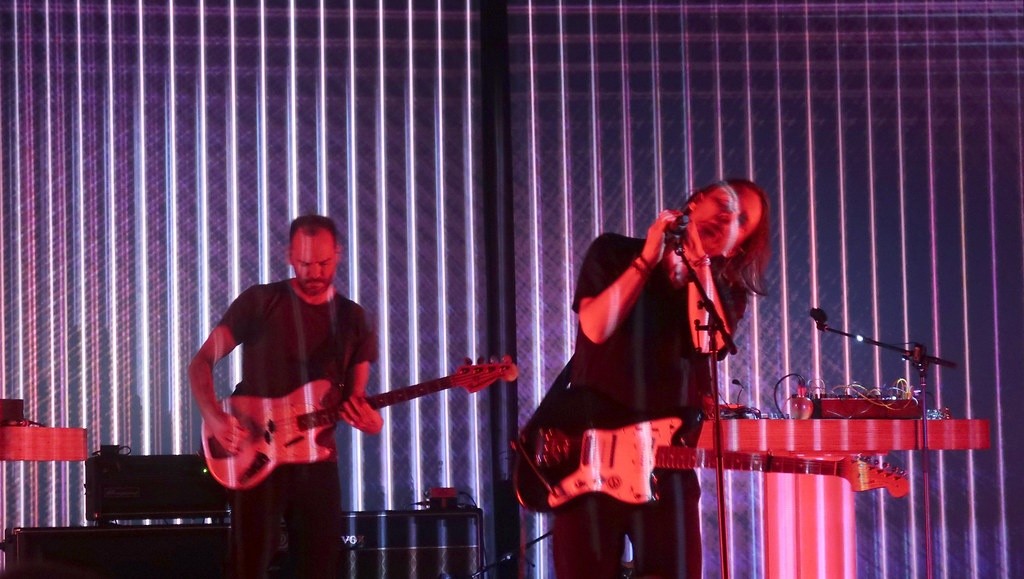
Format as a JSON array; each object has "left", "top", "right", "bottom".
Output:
[{"left": 632, "top": 255, "right": 651, "bottom": 278}]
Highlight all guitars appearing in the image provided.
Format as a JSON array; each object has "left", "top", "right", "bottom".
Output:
[
  {"left": 514, "top": 396, "right": 913, "bottom": 516},
  {"left": 200, "top": 353, "right": 520, "bottom": 492}
]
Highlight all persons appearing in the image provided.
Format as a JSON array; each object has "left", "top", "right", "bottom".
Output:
[
  {"left": 514, "top": 179, "right": 769, "bottom": 579},
  {"left": 187, "top": 215, "right": 383, "bottom": 579}
]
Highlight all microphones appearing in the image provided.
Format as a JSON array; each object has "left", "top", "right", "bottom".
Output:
[{"left": 665, "top": 214, "right": 690, "bottom": 241}]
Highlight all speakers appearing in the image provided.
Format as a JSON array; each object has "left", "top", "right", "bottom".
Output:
[
  {"left": 84, "top": 454, "right": 227, "bottom": 522},
  {"left": 4, "top": 523, "right": 233, "bottom": 579},
  {"left": 340, "top": 506, "right": 486, "bottom": 579}
]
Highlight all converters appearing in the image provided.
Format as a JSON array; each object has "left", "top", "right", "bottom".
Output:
[
  {"left": 100, "top": 445, "right": 121, "bottom": 455},
  {"left": 426, "top": 487, "right": 460, "bottom": 509}
]
